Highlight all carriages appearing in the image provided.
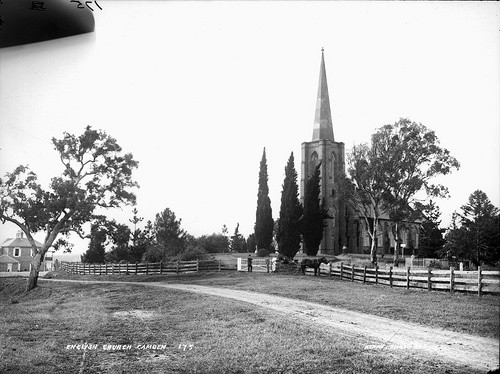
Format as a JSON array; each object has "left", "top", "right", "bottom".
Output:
[{"left": 271, "top": 254, "right": 328, "bottom": 276}]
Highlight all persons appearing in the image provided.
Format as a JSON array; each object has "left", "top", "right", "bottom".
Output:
[
  {"left": 248, "top": 254, "right": 253, "bottom": 272},
  {"left": 265, "top": 256, "right": 269, "bottom": 273}
]
